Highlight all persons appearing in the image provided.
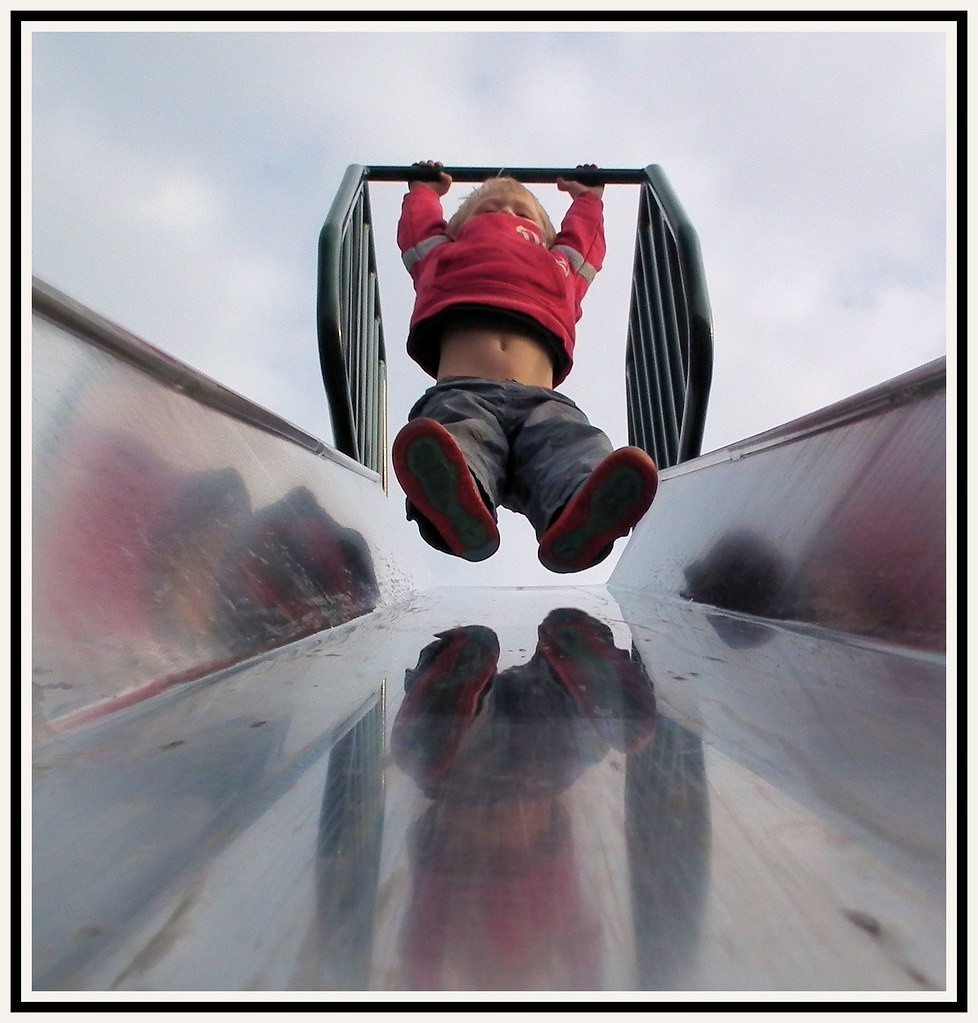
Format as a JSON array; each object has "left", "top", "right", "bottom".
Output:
[
  {"left": 392, "top": 606, "right": 659, "bottom": 992},
  {"left": 391, "top": 160, "right": 659, "bottom": 574}
]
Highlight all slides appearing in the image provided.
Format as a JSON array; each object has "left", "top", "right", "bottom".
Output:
[{"left": 31, "top": 274, "right": 946, "bottom": 990}]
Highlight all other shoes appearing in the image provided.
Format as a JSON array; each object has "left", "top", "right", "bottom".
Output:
[
  {"left": 540, "top": 607, "right": 657, "bottom": 754},
  {"left": 392, "top": 418, "right": 500, "bottom": 564},
  {"left": 387, "top": 624, "right": 500, "bottom": 781},
  {"left": 538, "top": 447, "right": 658, "bottom": 575}
]
